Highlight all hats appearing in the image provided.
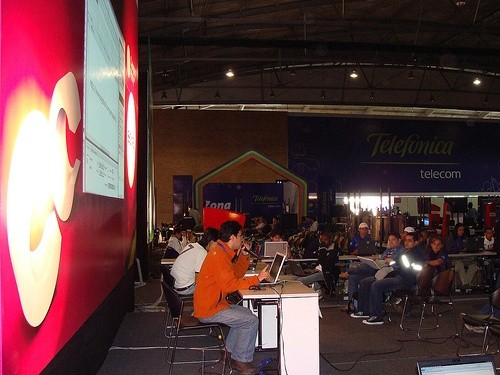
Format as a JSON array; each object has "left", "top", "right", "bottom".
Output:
[
  {"left": 358, "top": 222, "right": 369, "bottom": 230},
  {"left": 404, "top": 227, "right": 415, "bottom": 233}
]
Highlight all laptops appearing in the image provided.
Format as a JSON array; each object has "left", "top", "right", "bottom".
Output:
[
  {"left": 357, "top": 255, "right": 389, "bottom": 269},
  {"left": 259, "top": 252, "right": 286, "bottom": 284}
]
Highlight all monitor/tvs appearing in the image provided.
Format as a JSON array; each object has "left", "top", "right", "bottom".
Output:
[
  {"left": 416, "top": 217, "right": 430, "bottom": 227},
  {"left": 416, "top": 356, "right": 498, "bottom": 375}
]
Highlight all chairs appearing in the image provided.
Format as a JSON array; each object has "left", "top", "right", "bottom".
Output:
[
  {"left": 325, "top": 268, "right": 500, "bottom": 357},
  {"left": 160, "top": 265, "right": 233, "bottom": 375}
]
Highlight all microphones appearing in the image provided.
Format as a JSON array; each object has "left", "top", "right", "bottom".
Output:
[{"left": 241, "top": 244, "right": 262, "bottom": 261}]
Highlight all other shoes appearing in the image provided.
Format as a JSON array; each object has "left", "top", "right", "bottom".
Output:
[
  {"left": 337, "top": 304, "right": 356, "bottom": 312},
  {"left": 210, "top": 326, "right": 223, "bottom": 336},
  {"left": 333, "top": 288, "right": 349, "bottom": 297},
  {"left": 484, "top": 281, "right": 489, "bottom": 288},
  {"left": 219, "top": 351, "right": 234, "bottom": 369},
  {"left": 420, "top": 287, "right": 433, "bottom": 302},
  {"left": 431, "top": 292, "right": 441, "bottom": 308},
  {"left": 455, "top": 287, "right": 472, "bottom": 294},
  {"left": 464, "top": 320, "right": 492, "bottom": 335},
  {"left": 231, "top": 359, "right": 256, "bottom": 374}
]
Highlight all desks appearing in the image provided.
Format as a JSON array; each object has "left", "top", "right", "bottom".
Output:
[
  {"left": 237, "top": 270, "right": 320, "bottom": 375},
  {"left": 448, "top": 251, "right": 497, "bottom": 292},
  {"left": 161, "top": 258, "right": 177, "bottom": 264}
]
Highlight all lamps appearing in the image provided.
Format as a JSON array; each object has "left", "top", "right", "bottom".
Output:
[
  {"left": 350, "top": 69, "right": 358, "bottom": 78},
  {"left": 225, "top": 68, "right": 234, "bottom": 77}
]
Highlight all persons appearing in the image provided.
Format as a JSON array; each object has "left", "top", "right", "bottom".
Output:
[
  {"left": 170, "top": 227, "right": 231, "bottom": 350},
  {"left": 162, "top": 203, "right": 202, "bottom": 284},
  {"left": 193, "top": 220, "right": 270, "bottom": 375},
  {"left": 242, "top": 202, "right": 500, "bottom": 325}
]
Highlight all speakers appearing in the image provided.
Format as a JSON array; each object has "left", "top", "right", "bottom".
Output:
[{"left": 280, "top": 214, "right": 297, "bottom": 236}]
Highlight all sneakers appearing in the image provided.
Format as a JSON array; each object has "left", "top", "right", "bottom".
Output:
[
  {"left": 350, "top": 311, "right": 370, "bottom": 318},
  {"left": 362, "top": 315, "right": 385, "bottom": 325}
]
